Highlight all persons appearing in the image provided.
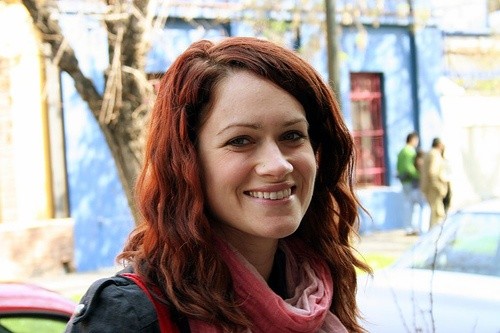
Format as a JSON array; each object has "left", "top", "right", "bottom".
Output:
[
  {"left": 421, "top": 137, "right": 449, "bottom": 231},
  {"left": 395, "top": 133, "right": 421, "bottom": 236},
  {"left": 64, "top": 36, "right": 374, "bottom": 333}
]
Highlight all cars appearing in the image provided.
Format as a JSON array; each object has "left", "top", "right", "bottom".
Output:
[{"left": 354, "top": 200, "right": 499, "bottom": 333}]
